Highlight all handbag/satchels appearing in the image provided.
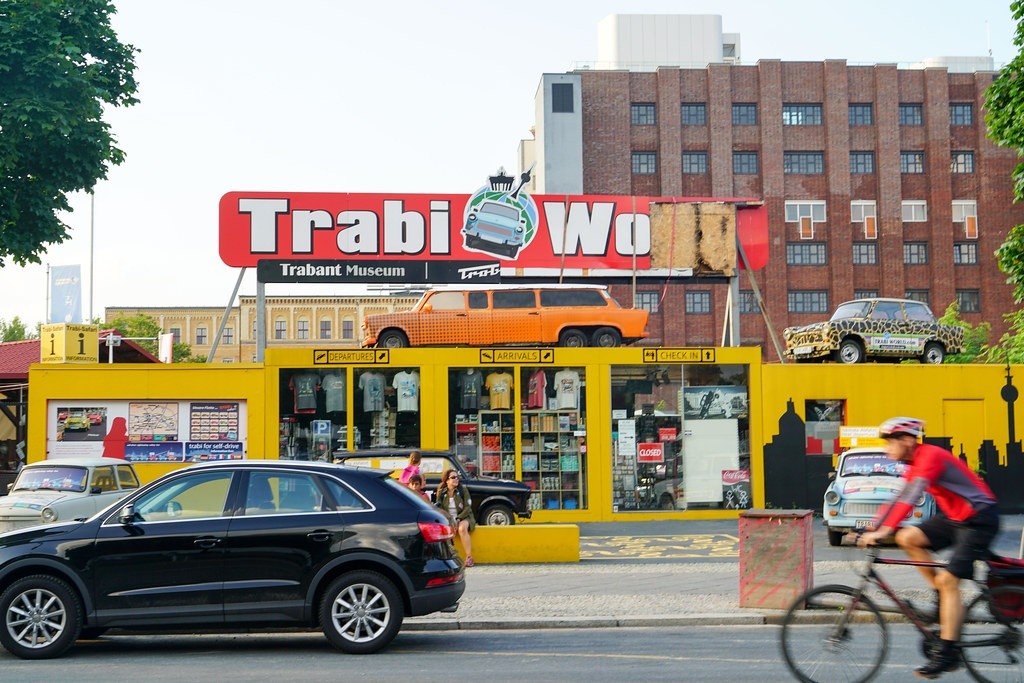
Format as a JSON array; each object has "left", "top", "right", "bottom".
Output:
[{"left": 986, "top": 557, "right": 1024, "bottom": 632}]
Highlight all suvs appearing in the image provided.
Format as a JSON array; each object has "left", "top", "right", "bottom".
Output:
[{"left": 0, "top": 457, "right": 467, "bottom": 663}]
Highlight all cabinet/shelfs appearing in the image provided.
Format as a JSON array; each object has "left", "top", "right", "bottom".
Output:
[
  {"left": 337, "top": 426, "right": 360, "bottom": 451},
  {"left": 478, "top": 410, "right": 583, "bottom": 509},
  {"left": 455, "top": 420, "right": 478, "bottom": 474}
]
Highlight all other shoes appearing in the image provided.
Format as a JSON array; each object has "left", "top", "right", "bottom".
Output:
[
  {"left": 925, "top": 589, "right": 940, "bottom": 625},
  {"left": 466, "top": 558, "right": 474, "bottom": 566},
  {"left": 913, "top": 642, "right": 960, "bottom": 678}
]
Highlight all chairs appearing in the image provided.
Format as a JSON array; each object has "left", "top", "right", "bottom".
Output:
[{"left": 246, "top": 476, "right": 276, "bottom": 515}]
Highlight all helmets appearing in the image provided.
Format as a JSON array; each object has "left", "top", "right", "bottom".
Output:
[{"left": 879, "top": 416, "right": 927, "bottom": 438}]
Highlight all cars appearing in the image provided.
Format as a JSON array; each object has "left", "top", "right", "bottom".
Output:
[
  {"left": 87, "top": 411, "right": 102, "bottom": 426},
  {"left": 63, "top": 412, "right": 90, "bottom": 431},
  {"left": 0, "top": 456, "right": 143, "bottom": 539},
  {"left": 361, "top": 282, "right": 652, "bottom": 347},
  {"left": 655, "top": 451, "right": 752, "bottom": 510},
  {"left": 822, "top": 446, "right": 936, "bottom": 546},
  {"left": 781, "top": 295, "right": 966, "bottom": 364},
  {"left": 329, "top": 449, "right": 534, "bottom": 526}
]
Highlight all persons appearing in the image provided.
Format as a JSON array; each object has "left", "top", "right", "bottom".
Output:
[
  {"left": 846, "top": 416, "right": 998, "bottom": 677},
  {"left": 437, "top": 470, "right": 475, "bottom": 567},
  {"left": 402, "top": 451, "right": 426, "bottom": 486},
  {"left": 289, "top": 368, "right": 420, "bottom": 413},
  {"left": 458, "top": 368, "right": 581, "bottom": 410},
  {"left": 408, "top": 475, "right": 429, "bottom": 501}
]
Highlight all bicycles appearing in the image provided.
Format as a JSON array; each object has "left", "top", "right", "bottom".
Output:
[{"left": 776, "top": 526, "right": 1024, "bottom": 683}]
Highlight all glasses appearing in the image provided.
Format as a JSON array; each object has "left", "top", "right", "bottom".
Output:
[{"left": 448, "top": 476, "right": 459, "bottom": 479}]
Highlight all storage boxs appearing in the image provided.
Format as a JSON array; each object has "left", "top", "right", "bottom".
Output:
[
  {"left": 522, "top": 455, "right": 537, "bottom": 470},
  {"left": 521, "top": 416, "right": 579, "bottom": 471},
  {"left": 545, "top": 498, "right": 559, "bottom": 509},
  {"left": 564, "top": 497, "right": 576, "bottom": 509}
]
